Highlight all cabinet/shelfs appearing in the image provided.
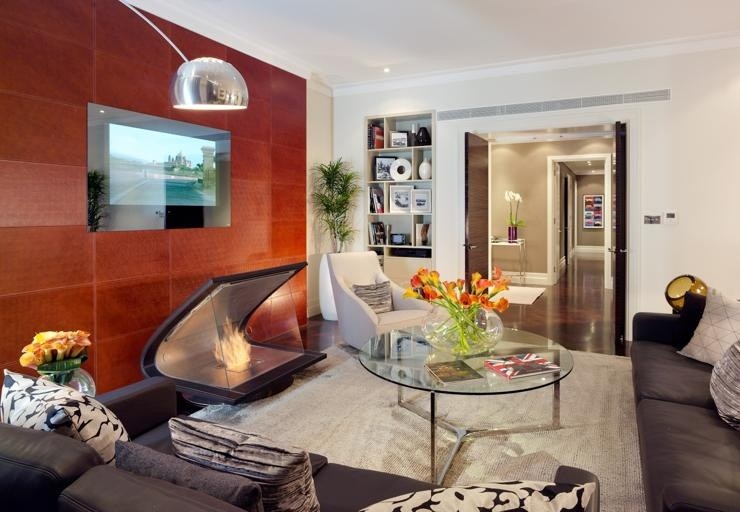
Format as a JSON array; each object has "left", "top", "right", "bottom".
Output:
[{"left": 363, "top": 109, "right": 437, "bottom": 288}]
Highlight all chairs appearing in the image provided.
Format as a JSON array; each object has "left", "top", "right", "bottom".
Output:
[{"left": 326, "top": 250, "right": 434, "bottom": 358}]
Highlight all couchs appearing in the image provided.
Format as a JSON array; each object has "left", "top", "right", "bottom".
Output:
[
  {"left": 0, "top": 376, "right": 599, "bottom": 512},
  {"left": 630, "top": 299, "right": 740, "bottom": 512}
]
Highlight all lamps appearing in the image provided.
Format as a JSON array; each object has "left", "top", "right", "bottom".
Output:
[
  {"left": 665, "top": 275, "right": 708, "bottom": 312},
  {"left": 115, "top": 0, "right": 250, "bottom": 111}
]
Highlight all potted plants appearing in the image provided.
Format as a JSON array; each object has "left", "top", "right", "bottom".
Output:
[{"left": 309, "top": 155, "right": 366, "bottom": 321}]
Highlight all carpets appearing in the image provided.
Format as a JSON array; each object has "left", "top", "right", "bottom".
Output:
[
  {"left": 488, "top": 284, "right": 546, "bottom": 305},
  {"left": 188, "top": 340, "right": 647, "bottom": 512}
]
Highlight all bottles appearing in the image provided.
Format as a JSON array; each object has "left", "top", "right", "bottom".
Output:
[
  {"left": 417, "top": 126, "right": 430, "bottom": 146},
  {"left": 418, "top": 157, "right": 432, "bottom": 179}
]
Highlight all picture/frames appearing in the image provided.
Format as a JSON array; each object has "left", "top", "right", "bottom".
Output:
[
  {"left": 389, "top": 185, "right": 414, "bottom": 213},
  {"left": 369, "top": 221, "right": 384, "bottom": 245},
  {"left": 389, "top": 130, "right": 408, "bottom": 148},
  {"left": 411, "top": 189, "right": 432, "bottom": 213},
  {"left": 582, "top": 194, "right": 604, "bottom": 229},
  {"left": 374, "top": 155, "right": 397, "bottom": 181}
]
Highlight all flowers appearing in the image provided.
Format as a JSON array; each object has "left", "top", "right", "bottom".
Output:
[
  {"left": 19, "top": 330, "right": 93, "bottom": 384},
  {"left": 403, "top": 267, "right": 512, "bottom": 355},
  {"left": 504, "top": 190, "right": 528, "bottom": 228}
]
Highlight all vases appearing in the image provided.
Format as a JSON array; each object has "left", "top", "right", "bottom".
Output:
[
  {"left": 508, "top": 227, "right": 517, "bottom": 244},
  {"left": 420, "top": 303, "right": 505, "bottom": 355},
  {"left": 37, "top": 358, "right": 96, "bottom": 397}
]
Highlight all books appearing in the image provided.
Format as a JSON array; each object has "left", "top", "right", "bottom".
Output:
[
  {"left": 368, "top": 124, "right": 385, "bottom": 245},
  {"left": 484, "top": 353, "right": 559, "bottom": 379},
  {"left": 422, "top": 360, "right": 485, "bottom": 385}
]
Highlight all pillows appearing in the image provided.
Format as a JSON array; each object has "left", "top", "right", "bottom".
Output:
[
  {"left": 675, "top": 286, "right": 740, "bottom": 367},
  {"left": 0, "top": 368, "right": 132, "bottom": 470},
  {"left": 168, "top": 415, "right": 321, "bottom": 512},
  {"left": 709, "top": 340, "right": 740, "bottom": 432},
  {"left": 115, "top": 439, "right": 265, "bottom": 512},
  {"left": 358, "top": 480, "right": 596, "bottom": 512},
  {"left": 351, "top": 281, "right": 395, "bottom": 315}
]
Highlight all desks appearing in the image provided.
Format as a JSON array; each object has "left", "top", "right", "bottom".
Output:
[{"left": 491, "top": 239, "right": 527, "bottom": 287}]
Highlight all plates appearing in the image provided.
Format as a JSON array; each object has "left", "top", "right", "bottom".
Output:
[{"left": 390, "top": 159, "right": 412, "bottom": 181}]
